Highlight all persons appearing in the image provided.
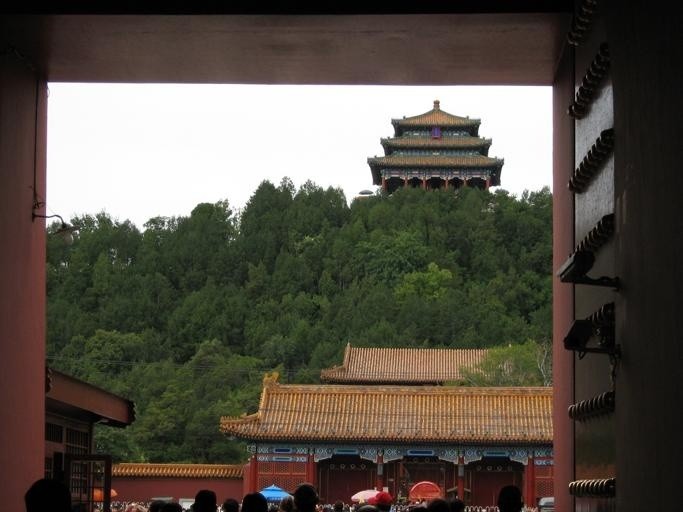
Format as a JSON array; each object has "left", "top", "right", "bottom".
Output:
[
  {"left": 149, "top": 485, "right": 465, "bottom": 511},
  {"left": 24, "top": 478, "right": 72, "bottom": 511},
  {"left": 497, "top": 485, "right": 522, "bottom": 511}
]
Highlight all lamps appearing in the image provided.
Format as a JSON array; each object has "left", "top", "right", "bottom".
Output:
[{"left": 94, "top": 418, "right": 109, "bottom": 425}]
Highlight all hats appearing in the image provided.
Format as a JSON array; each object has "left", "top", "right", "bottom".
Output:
[{"left": 366, "top": 492, "right": 395, "bottom": 505}]
